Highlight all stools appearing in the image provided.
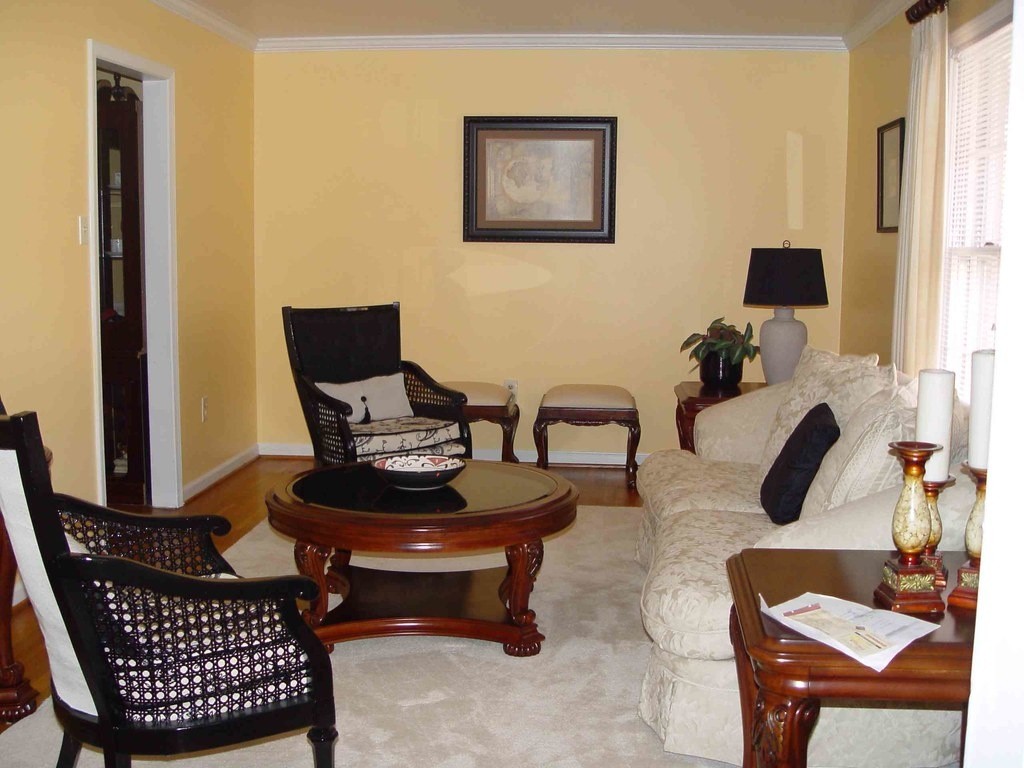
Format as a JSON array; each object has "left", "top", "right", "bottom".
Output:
[
  {"left": 534, "top": 383, "right": 641, "bottom": 487},
  {"left": 412, "top": 382, "right": 519, "bottom": 465}
]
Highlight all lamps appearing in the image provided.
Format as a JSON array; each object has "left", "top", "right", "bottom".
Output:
[{"left": 743, "top": 240, "right": 829, "bottom": 385}]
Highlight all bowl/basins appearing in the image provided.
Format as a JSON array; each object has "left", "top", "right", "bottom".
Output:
[{"left": 370, "top": 453, "right": 467, "bottom": 491}]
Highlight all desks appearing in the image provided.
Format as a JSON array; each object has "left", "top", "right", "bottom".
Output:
[
  {"left": 0, "top": 449, "right": 52, "bottom": 726},
  {"left": 726, "top": 547, "right": 983, "bottom": 768},
  {"left": 675, "top": 381, "right": 768, "bottom": 456}
]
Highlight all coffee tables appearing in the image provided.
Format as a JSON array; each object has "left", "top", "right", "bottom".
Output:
[{"left": 264, "top": 458, "right": 580, "bottom": 657}]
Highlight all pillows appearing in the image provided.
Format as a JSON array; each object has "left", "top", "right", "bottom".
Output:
[
  {"left": 314, "top": 372, "right": 414, "bottom": 423},
  {"left": 65, "top": 535, "right": 144, "bottom": 659},
  {"left": 760, "top": 347, "right": 961, "bottom": 525}
]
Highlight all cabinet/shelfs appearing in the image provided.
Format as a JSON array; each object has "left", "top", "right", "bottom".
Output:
[{"left": 95, "top": 72, "right": 152, "bottom": 507}]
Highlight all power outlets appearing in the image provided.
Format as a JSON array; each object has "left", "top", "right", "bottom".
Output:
[
  {"left": 504, "top": 380, "right": 518, "bottom": 401},
  {"left": 202, "top": 396, "right": 207, "bottom": 421}
]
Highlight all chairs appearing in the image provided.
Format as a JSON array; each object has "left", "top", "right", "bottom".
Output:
[
  {"left": 281, "top": 300, "right": 473, "bottom": 466},
  {"left": 0, "top": 411, "right": 338, "bottom": 768}
]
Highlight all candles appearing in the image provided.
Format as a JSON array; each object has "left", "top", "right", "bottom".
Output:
[
  {"left": 913, "top": 367, "right": 953, "bottom": 481},
  {"left": 968, "top": 348, "right": 995, "bottom": 470}
]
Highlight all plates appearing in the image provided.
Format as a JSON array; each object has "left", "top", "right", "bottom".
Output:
[
  {"left": 108, "top": 184, "right": 121, "bottom": 189},
  {"left": 105, "top": 252, "right": 123, "bottom": 257}
]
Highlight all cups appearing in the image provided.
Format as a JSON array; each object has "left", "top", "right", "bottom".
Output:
[
  {"left": 111, "top": 239, "right": 122, "bottom": 252},
  {"left": 114, "top": 172, "right": 121, "bottom": 183}
]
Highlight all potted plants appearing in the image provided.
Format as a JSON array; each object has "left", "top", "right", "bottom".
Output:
[{"left": 680, "top": 317, "right": 761, "bottom": 389}]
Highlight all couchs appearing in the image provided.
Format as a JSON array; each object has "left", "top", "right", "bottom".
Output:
[{"left": 637, "top": 367, "right": 980, "bottom": 767}]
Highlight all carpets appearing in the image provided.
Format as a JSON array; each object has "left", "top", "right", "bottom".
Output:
[{"left": 0, "top": 505, "right": 738, "bottom": 768}]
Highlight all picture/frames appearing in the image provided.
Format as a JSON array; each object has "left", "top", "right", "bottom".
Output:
[
  {"left": 463, "top": 116, "right": 617, "bottom": 243},
  {"left": 876, "top": 116, "right": 905, "bottom": 233}
]
